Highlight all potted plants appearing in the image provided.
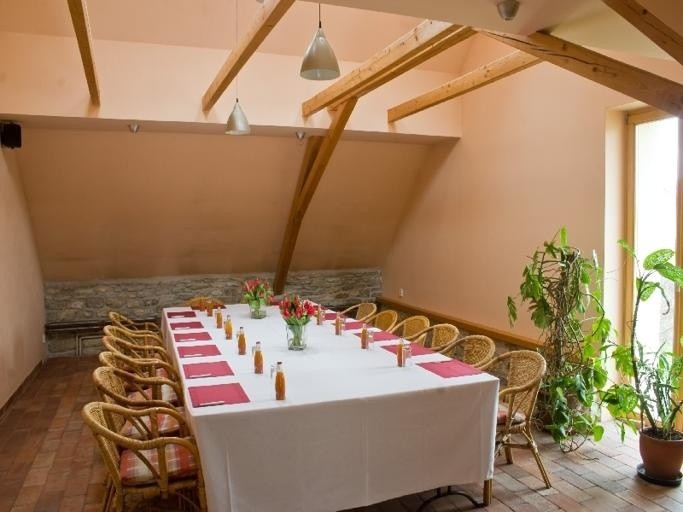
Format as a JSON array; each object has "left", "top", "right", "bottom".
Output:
[
  {"left": 503, "top": 226, "right": 637, "bottom": 461},
  {"left": 600, "top": 239, "right": 683, "bottom": 486}
]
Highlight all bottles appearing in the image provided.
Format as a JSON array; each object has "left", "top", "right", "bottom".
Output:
[
  {"left": 361, "top": 324, "right": 369, "bottom": 348},
  {"left": 396, "top": 336, "right": 405, "bottom": 366},
  {"left": 253, "top": 340, "right": 264, "bottom": 374},
  {"left": 274, "top": 361, "right": 285, "bottom": 400},
  {"left": 237, "top": 326, "right": 246, "bottom": 355},
  {"left": 198, "top": 295, "right": 232, "bottom": 340},
  {"left": 316, "top": 303, "right": 342, "bottom": 336}
]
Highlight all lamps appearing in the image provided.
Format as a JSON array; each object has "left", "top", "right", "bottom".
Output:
[
  {"left": 298, "top": 2, "right": 341, "bottom": 81},
  {"left": 223, "top": 1, "right": 250, "bottom": 140}
]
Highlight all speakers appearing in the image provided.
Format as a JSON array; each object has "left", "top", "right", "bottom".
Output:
[{"left": 0, "top": 123, "right": 22, "bottom": 149}]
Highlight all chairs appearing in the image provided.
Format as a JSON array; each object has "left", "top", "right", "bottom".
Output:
[
  {"left": 342, "top": 303, "right": 377, "bottom": 322},
  {"left": 98, "top": 349, "right": 182, "bottom": 405},
  {"left": 476, "top": 349, "right": 552, "bottom": 492},
  {"left": 402, "top": 324, "right": 460, "bottom": 352},
  {"left": 439, "top": 335, "right": 497, "bottom": 367},
  {"left": 390, "top": 314, "right": 430, "bottom": 346},
  {"left": 100, "top": 333, "right": 175, "bottom": 380},
  {"left": 79, "top": 395, "right": 207, "bottom": 510},
  {"left": 89, "top": 367, "right": 185, "bottom": 440},
  {"left": 98, "top": 321, "right": 166, "bottom": 356},
  {"left": 365, "top": 310, "right": 397, "bottom": 332},
  {"left": 104, "top": 307, "right": 160, "bottom": 337}
]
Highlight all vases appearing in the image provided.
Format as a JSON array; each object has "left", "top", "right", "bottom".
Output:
[
  {"left": 285, "top": 325, "right": 308, "bottom": 351},
  {"left": 248, "top": 298, "right": 268, "bottom": 320}
]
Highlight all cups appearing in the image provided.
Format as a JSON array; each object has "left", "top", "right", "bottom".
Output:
[
  {"left": 366, "top": 334, "right": 373, "bottom": 351},
  {"left": 401, "top": 345, "right": 410, "bottom": 366},
  {"left": 251, "top": 345, "right": 255, "bottom": 368},
  {"left": 270, "top": 364, "right": 276, "bottom": 381}
]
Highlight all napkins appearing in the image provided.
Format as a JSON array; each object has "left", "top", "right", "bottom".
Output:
[
  {"left": 380, "top": 342, "right": 435, "bottom": 357},
  {"left": 355, "top": 332, "right": 399, "bottom": 342},
  {"left": 417, "top": 359, "right": 483, "bottom": 379},
  {"left": 333, "top": 322, "right": 369, "bottom": 332}
]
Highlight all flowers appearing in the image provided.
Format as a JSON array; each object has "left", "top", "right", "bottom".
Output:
[
  {"left": 241, "top": 278, "right": 272, "bottom": 300},
  {"left": 278, "top": 293, "right": 318, "bottom": 344}
]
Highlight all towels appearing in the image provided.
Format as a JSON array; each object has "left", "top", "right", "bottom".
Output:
[
  {"left": 165, "top": 304, "right": 250, "bottom": 409},
  {"left": 314, "top": 310, "right": 343, "bottom": 322}
]
